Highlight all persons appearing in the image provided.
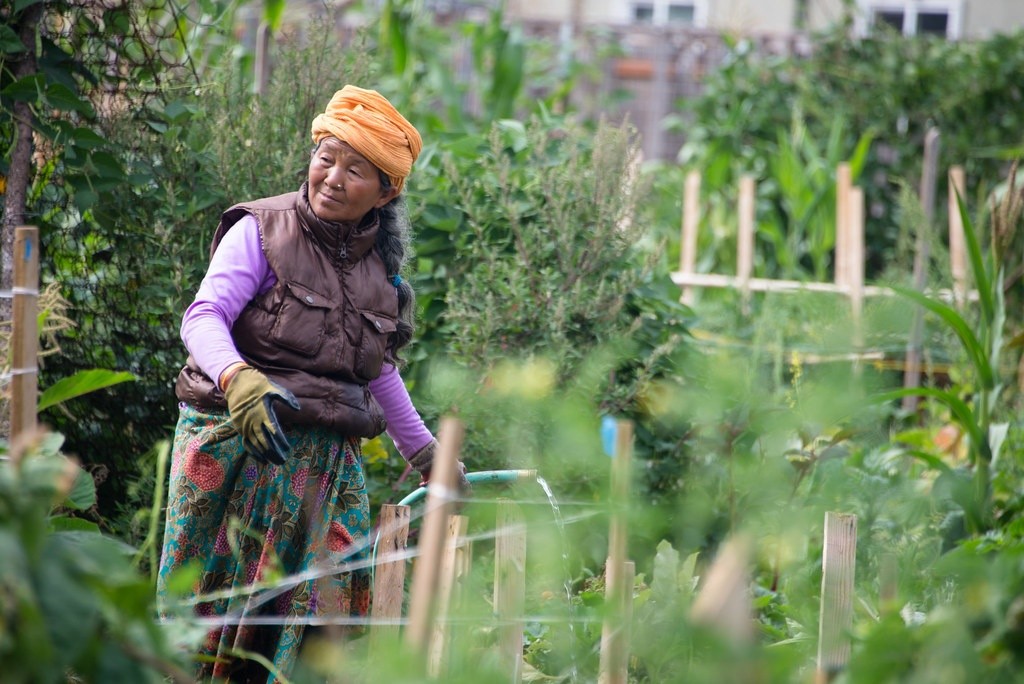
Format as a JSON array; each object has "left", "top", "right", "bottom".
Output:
[{"left": 145, "top": 83, "right": 473, "bottom": 684}]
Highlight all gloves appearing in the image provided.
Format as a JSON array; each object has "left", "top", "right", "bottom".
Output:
[
  {"left": 224, "top": 366, "right": 300, "bottom": 465},
  {"left": 408, "top": 437, "right": 474, "bottom": 515}
]
